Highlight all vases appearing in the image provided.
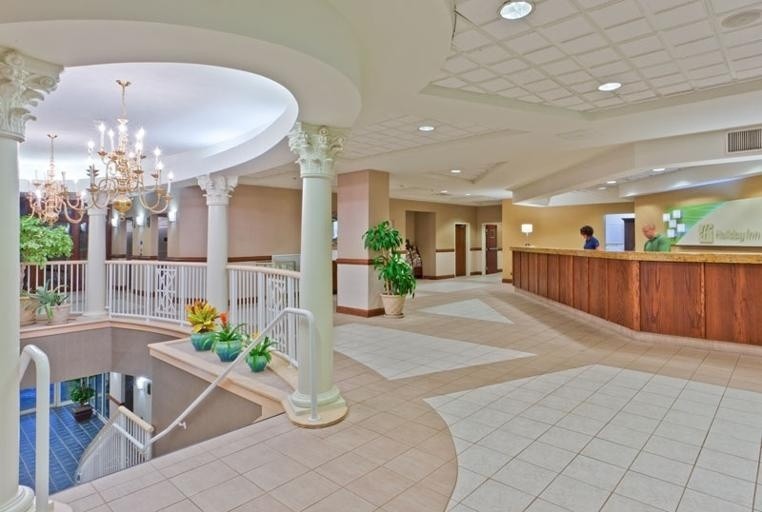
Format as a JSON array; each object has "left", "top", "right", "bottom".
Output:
[{"left": 215, "top": 340, "right": 241, "bottom": 362}]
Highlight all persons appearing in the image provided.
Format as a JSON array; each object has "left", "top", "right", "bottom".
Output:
[
  {"left": 580, "top": 225, "right": 604, "bottom": 252},
  {"left": 641, "top": 221, "right": 672, "bottom": 253}
]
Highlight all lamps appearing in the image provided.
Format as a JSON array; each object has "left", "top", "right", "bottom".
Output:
[
  {"left": 87, "top": 77, "right": 174, "bottom": 222},
  {"left": 25, "top": 124, "right": 86, "bottom": 225},
  {"left": 521, "top": 224, "right": 533, "bottom": 246}
]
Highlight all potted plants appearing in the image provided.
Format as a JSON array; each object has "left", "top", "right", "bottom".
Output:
[
  {"left": 20, "top": 219, "right": 74, "bottom": 322},
  {"left": 361, "top": 219, "right": 419, "bottom": 323},
  {"left": 30, "top": 280, "right": 73, "bottom": 323},
  {"left": 70, "top": 386, "right": 96, "bottom": 421},
  {"left": 185, "top": 297, "right": 221, "bottom": 352},
  {"left": 239, "top": 332, "right": 272, "bottom": 370}
]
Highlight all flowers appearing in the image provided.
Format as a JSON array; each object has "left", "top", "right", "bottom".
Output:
[{"left": 198, "top": 312, "right": 249, "bottom": 353}]
